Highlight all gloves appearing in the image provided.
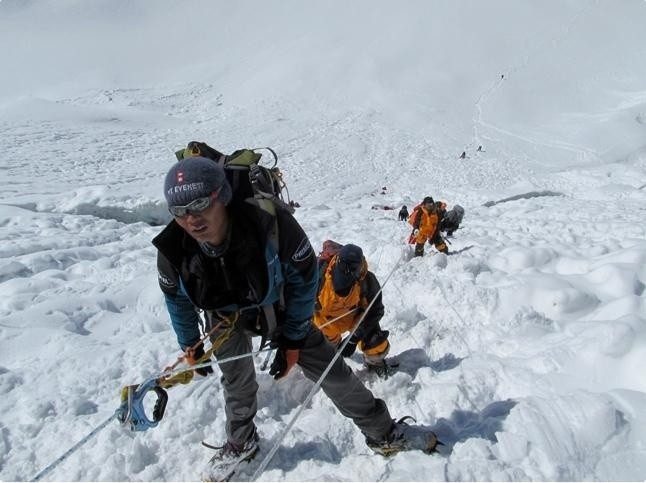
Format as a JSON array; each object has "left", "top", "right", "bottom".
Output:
[
  {"left": 337, "top": 333, "right": 359, "bottom": 358},
  {"left": 180, "top": 342, "right": 214, "bottom": 377},
  {"left": 268, "top": 334, "right": 301, "bottom": 382}
]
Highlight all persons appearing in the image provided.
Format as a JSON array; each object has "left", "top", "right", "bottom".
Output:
[
  {"left": 436, "top": 203, "right": 447, "bottom": 219},
  {"left": 312, "top": 244, "right": 394, "bottom": 381},
  {"left": 149, "top": 158, "right": 437, "bottom": 481},
  {"left": 438, "top": 217, "right": 460, "bottom": 237},
  {"left": 398, "top": 205, "right": 410, "bottom": 220},
  {"left": 407, "top": 196, "right": 450, "bottom": 257}
]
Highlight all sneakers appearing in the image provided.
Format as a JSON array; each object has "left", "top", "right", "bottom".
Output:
[
  {"left": 362, "top": 422, "right": 439, "bottom": 456},
  {"left": 365, "top": 359, "right": 395, "bottom": 383},
  {"left": 198, "top": 426, "right": 262, "bottom": 482}
]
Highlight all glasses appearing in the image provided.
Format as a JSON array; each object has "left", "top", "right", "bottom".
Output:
[{"left": 168, "top": 184, "right": 224, "bottom": 217}]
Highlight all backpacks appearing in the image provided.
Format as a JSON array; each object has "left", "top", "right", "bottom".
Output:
[
  {"left": 174, "top": 140, "right": 301, "bottom": 254},
  {"left": 317, "top": 239, "right": 344, "bottom": 261}
]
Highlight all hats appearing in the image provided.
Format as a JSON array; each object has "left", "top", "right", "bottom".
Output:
[
  {"left": 336, "top": 243, "right": 364, "bottom": 272},
  {"left": 162, "top": 155, "right": 233, "bottom": 218},
  {"left": 423, "top": 196, "right": 446, "bottom": 209}
]
